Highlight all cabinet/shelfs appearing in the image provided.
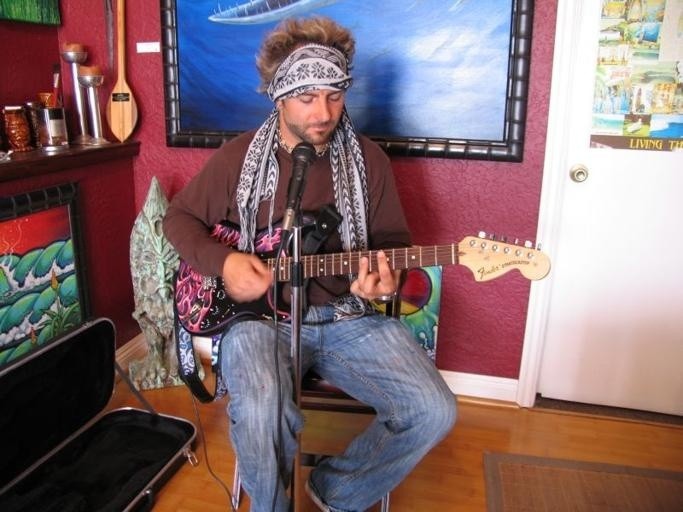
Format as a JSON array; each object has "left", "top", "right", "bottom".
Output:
[{"left": 0, "top": 141, "right": 142, "bottom": 350}]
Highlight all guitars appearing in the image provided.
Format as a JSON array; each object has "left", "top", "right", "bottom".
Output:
[{"left": 174, "top": 210, "right": 550, "bottom": 336}]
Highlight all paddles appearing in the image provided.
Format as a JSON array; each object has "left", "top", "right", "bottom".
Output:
[{"left": 107, "top": 0, "right": 140, "bottom": 145}]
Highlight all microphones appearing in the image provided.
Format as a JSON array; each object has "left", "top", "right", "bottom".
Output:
[{"left": 280, "top": 141, "right": 316, "bottom": 236}]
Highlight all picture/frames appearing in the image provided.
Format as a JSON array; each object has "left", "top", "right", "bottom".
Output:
[{"left": 160, "top": 1, "right": 534, "bottom": 162}]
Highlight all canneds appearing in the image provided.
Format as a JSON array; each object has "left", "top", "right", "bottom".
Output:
[{"left": 0, "top": 101, "right": 44, "bottom": 153}]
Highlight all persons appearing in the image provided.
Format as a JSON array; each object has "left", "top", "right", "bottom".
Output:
[{"left": 161, "top": 13, "right": 458, "bottom": 511}]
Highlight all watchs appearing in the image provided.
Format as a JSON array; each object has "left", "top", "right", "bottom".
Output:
[{"left": 374, "top": 292, "right": 397, "bottom": 305}]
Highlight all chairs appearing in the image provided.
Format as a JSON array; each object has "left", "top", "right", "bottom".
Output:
[{"left": 232, "top": 293, "right": 402, "bottom": 510}]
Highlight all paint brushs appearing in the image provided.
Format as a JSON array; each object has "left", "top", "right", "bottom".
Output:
[{"left": 52, "top": 63, "right": 61, "bottom": 109}]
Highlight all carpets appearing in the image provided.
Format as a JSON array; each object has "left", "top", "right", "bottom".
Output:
[{"left": 482, "top": 448, "right": 682, "bottom": 512}]
